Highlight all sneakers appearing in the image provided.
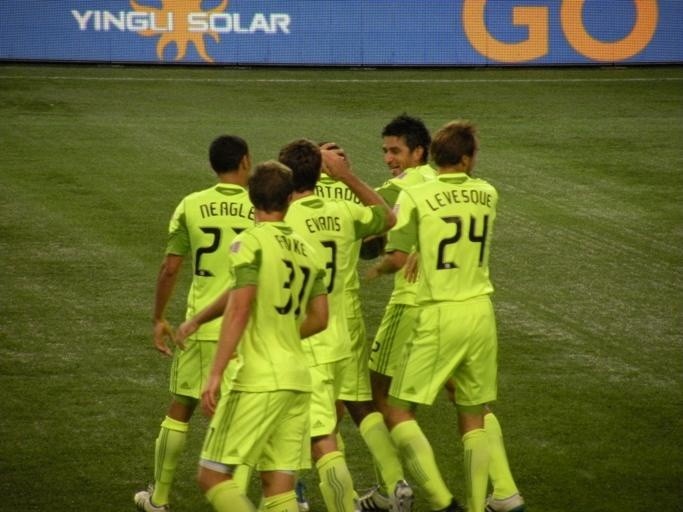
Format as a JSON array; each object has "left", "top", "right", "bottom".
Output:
[
  {"left": 485, "top": 495, "right": 526, "bottom": 512},
  {"left": 389, "top": 480, "right": 413, "bottom": 512},
  {"left": 359, "top": 485, "right": 389, "bottom": 510},
  {"left": 134, "top": 492, "right": 170, "bottom": 512}
]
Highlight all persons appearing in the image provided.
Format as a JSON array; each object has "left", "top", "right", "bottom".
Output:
[
  {"left": 358, "top": 112, "right": 526, "bottom": 512},
  {"left": 176, "top": 139, "right": 414, "bottom": 512},
  {"left": 132, "top": 135, "right": 257, "bottom": 511},
  {"left": 201, "top": 160, "right": 330, "bottom": 512},
  {"left": 368, "top": 116, "right": 499, "bottom": 512},
  {"left": 295, "top": 143, "right": 393, "bottom": 512}
]
